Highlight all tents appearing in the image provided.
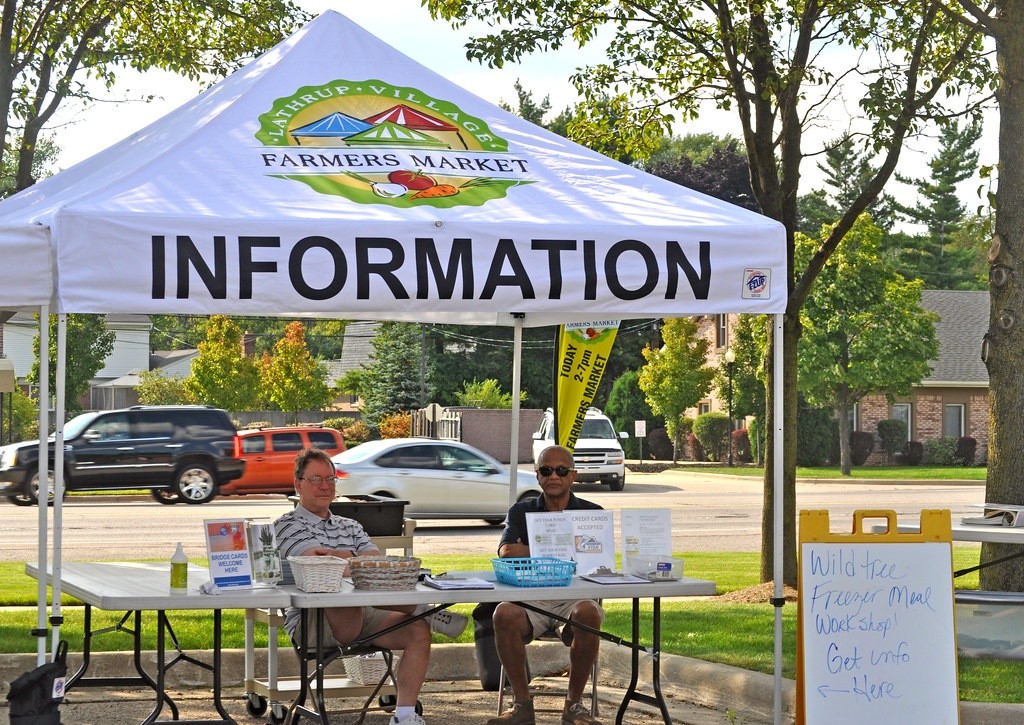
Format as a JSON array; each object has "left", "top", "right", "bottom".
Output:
[{"left": 0, "top": 9, "right": 788, "bottom": 724}]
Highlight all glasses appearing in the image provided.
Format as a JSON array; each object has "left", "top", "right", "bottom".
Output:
[
  {"left": 532, "top": 464, "right": 576, "bottom": 477},
  {"left": 296, "top": 475, "right": 341, "bottom": 484}
]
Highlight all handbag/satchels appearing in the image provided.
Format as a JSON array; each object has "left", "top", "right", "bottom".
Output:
[{"left": 7, "top": 640, "right": 69, "bottom": 725}]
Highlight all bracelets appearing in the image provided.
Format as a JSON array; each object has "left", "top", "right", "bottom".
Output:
[{"left": 351, "top": 550, "right": 356, "bottom": 557}]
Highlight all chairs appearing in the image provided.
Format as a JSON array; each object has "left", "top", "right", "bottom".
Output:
[
  {"left": 497, "top": 598, "right": 602, "bottom": 719},
  {"left": 581, "top": 423, "right": 601, "bottom": 438},
  {"left": 279, "top": 608, "right": 398, "bottom": 725}
]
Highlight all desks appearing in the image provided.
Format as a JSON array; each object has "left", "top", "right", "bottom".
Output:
[
  {"left": 272, "top": 572, "right": 716, "bottom": 725},
  {"left": 25, "top": 561, "right": 290, "bottom": 725},
  {"left": 872, "top": 521, "right": 1024, "bottom": 578}
]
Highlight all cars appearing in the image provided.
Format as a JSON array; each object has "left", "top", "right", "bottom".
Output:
[{"left": 326, "top": 436, "right": 545, "bottom": 525}]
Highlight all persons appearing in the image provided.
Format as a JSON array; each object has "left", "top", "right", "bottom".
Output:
[
  {"left": 273, "top": 448, "right": 468, "bottom": 724},
  {"left": 104, "top": 417, "right": 127, "bottom": 440},
  {"left": 487, "top": 446, "right": 604, "bottom": 725}
]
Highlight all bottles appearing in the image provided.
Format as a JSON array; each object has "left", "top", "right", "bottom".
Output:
[{"left": 170, "top": 542, "right": 187, "bottom": 594}]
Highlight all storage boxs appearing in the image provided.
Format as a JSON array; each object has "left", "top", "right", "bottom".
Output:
[
  {"left": 627, "top": 554, "right": 684, "bottom": 581},
  {"left": 955, "top": 589, "right": 1024, "bottom": 660},
  {"left": 288, "top": 495, "right": 410, "bottom": 536}
]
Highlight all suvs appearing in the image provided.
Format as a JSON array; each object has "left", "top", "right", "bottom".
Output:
[
  {"left": 531, "top": 406, "right": 630, "bottom": 491},
  {"left": 0, "top": 403, "right": 249, "bottom": 508},
  {"left": 214, "top": 423, "right": 351, "bottom": 498}
]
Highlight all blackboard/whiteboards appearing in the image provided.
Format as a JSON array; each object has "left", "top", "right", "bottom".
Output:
[{"left": 802, "top": 543, "right": 957, "bottom": 720}]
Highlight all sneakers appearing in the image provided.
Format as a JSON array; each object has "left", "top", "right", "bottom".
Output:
[
  {"left": 561, "top": 694, "right": 603, "bottom": 725},
  {"left": 389, "top": 711, "right": 427, "bottom": 725},
  {"left": 485, "top": 692, "right": 537, "bottom": 725},
  {"left": 424, "top": 602, "right": 470, "bottom": 639}
]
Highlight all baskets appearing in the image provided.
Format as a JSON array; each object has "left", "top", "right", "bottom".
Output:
[
  {"left": 342, "top": 652, "right": 400, "bottom": 685},
  {"left": 491, "top": 555, "right": 579, "bottom": 589},
  {"left": 286, "top": 554, "right": 350, "bottom": 593},
  {"left": 344, "top": 554, "right": 422, "bottom": 591}
]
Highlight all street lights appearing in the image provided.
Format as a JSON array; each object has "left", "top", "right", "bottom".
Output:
[{"left": 725, "top": 350, "right": 736, "bottom": 468}]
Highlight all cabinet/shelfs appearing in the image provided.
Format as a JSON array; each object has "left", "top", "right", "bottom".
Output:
[{"left": 244, "top": 517, "right": 423, "bottom": 725}]
